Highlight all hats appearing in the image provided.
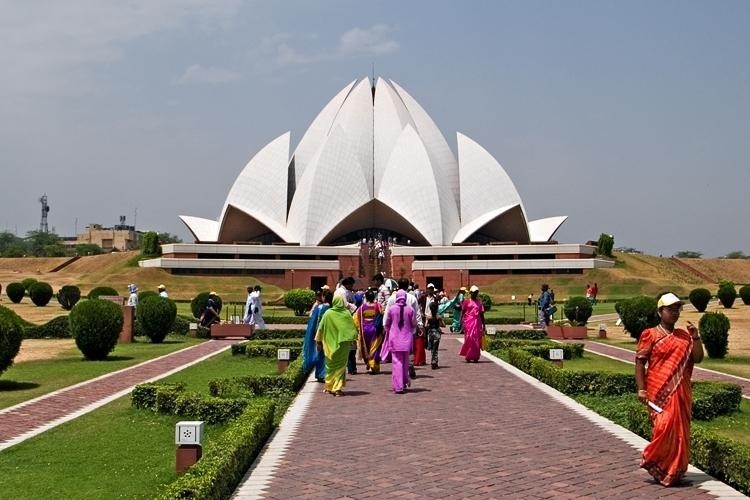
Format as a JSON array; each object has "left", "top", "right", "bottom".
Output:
[
  {"left": 427, "top": 283, "right": 434, "bottom": 288},
  {"left": 470, "top": 285, "right": 479, "bottom": 292},
  {"left": 459, "top": 287, "right": 468, "bottom": 293},
  {"left": 320, "top": 284, "right": 330, "bottom": 290},
  {"left": 657, "top": 293, "right": 681, "bottom": 310},
  {"left": 157, "top": 284, "right": 165, "bottom": 289},
  {"left": 209, "top": 291, "right": 218, "bottom": 296}
]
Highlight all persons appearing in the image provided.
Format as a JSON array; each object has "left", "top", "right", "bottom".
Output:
[
  {"left": 354, "top": 231, "right": 398, "bottom": 266},
  {"left": 242, "top": 286, "right": 254, "bottom": 324},
  {"left": 583, "top": 284, "right": 592, "bottom": 306},
  {"left": 527, "top": 293, "right": 533, "bottom": 306},
  {"left": 550, "top": 289, "right": 555, "bottom": 305},
  {"left": 633, "top": 291, "right": 705, "bottom": 488},
  {"left": 248, "top": 284, "right": 266, "bottom": 329},
  {"left": 537, "top": 283, "right": 554, "bottom": 329},
  {"left": 155, "top": 283, "right": 168, "bottom": 299},
  {"left": 199, "top": 291, "right": 221, "bottom": 327},
  {"left": 300, "top": 270, "right": 487, "bottom": 398},
  {"left": 591, "top": 282, "right": 599, "bottom": 305},
  {"left": 126, "top": 283, "right": 139, "bottom": 318}
]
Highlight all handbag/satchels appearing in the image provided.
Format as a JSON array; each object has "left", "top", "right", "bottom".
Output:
[
  {"left": 481, "top": 335, "right": 486, "bottom": 351},
  {"left": 545, "top": 306, "right": 557, "bottom": 316}
]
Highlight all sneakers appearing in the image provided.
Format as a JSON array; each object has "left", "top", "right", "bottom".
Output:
[{"left": 408, "top": 364, "right": 417, "bottom": 379}]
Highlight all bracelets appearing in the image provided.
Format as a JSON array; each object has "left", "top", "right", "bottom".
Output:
[
  {"left": 637, "top": 389, "right": 648, "bottom": 394},
  {"left": 690, "top": 337, "right": 701, "bottom": 341}
]
